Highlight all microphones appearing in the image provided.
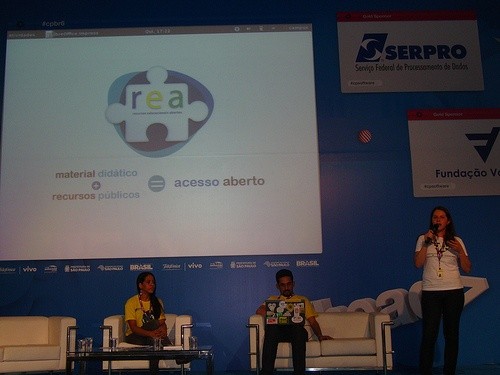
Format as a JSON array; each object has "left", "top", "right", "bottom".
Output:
[{"left": 426, "top": 224, "right": 438, "bottom": 244}]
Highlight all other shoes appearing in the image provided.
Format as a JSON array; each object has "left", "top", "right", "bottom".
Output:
[{"left": 176, "top": 358, "right": 195, "bottom": 365}]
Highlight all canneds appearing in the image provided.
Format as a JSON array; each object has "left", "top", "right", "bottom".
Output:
[
  {"left": 188, "top": 336, "right": 197, "bottom": 350},
  {"left": 110, "top": 338, "right": 117, "bottom": 351},
  {"left": 153, "top": 338, "right": 161, "bottom": 351},
  {"left": 78, "top": 337, "right": 92, "bottom": 352}
]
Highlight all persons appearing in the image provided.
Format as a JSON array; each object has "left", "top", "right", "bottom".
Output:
[
  {"left": 415, "top": 207, "right": 471, "bottom": 375},
  {"left": 125, "top": 272, "right": 194, "bottom": 370},
  {"left": 256, "top": 268, "right": 334, "bottom": 375}
]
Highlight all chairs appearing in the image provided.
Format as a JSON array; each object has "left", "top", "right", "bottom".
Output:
[
  {"left": 99, "top": 314, "right": 195, "bottom": 375},
  {"left": 0, "top": 316, "right": 80, "bottom": 375}
]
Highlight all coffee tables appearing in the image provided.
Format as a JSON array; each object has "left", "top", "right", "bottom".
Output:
[{"left": 67, "top": 346, "right": 214, "bottom": 375}]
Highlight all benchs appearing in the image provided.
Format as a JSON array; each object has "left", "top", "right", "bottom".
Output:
[{"left": 246, "top": 311, "right": 396, "bottom": 375}]
